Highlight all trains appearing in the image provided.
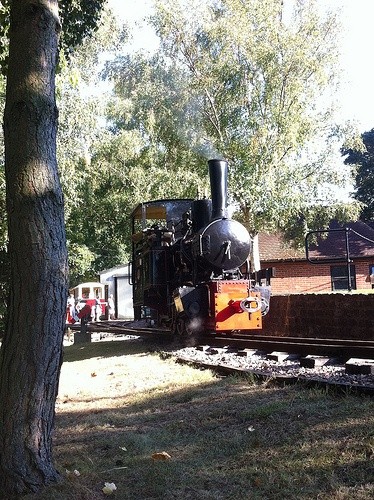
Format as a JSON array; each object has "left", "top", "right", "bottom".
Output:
[{"left": 129, "top": 159, "right": 262, "bottom": 336}]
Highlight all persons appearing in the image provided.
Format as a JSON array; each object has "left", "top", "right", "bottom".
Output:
[
  {"left": 165, "top": 220, "right": 175, "bottom": 246},
  {"left": 65, "top": 292, "right": 103, "bottom": 342},
  {"left": 108, "top": 293, "right": 116, "bottom": 321}
]
